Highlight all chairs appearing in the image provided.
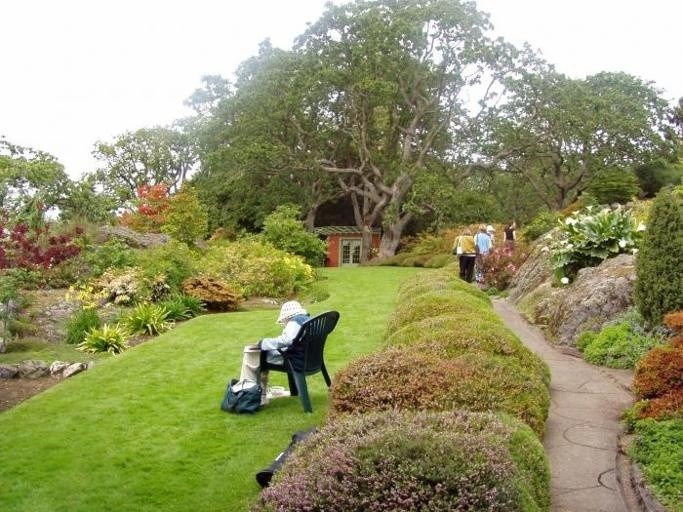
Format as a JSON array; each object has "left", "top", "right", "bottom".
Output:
[{"left": 261, "top": 310, "right": 340, "bottom": 413}]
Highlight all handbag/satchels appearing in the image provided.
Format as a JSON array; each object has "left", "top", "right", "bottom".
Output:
[
  {"left": 219, "top": 377, "right": 262, "bottom": 413},
  {"left": 475, "top": 245, "right": 480, "bottom": 255},
  {"left": 455, "top": 236, "right": 463, "bottom": 257}
]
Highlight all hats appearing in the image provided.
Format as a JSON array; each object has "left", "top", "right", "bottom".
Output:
[
  {"left": 487, "top": 225, "right": 497, "bottom": 232},
  {"left": 277, "top": 300, "right": 310, "bottom": 324}
]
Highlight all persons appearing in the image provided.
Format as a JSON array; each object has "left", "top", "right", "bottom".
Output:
[
  {"left": 238, "top": 301, "right": 311, "bottom": 407},
  {"left": 487, "top": 223, "right": 497, "bottom": 243},
  {"left": 503, "top": 219, "right": 517, "bottom": 256},
  {"left": 474, "top": 225, "right": 493, "bottom": 282},
  {"left": 451, "top": 228, "right": 478, "bottom": 284}
]
